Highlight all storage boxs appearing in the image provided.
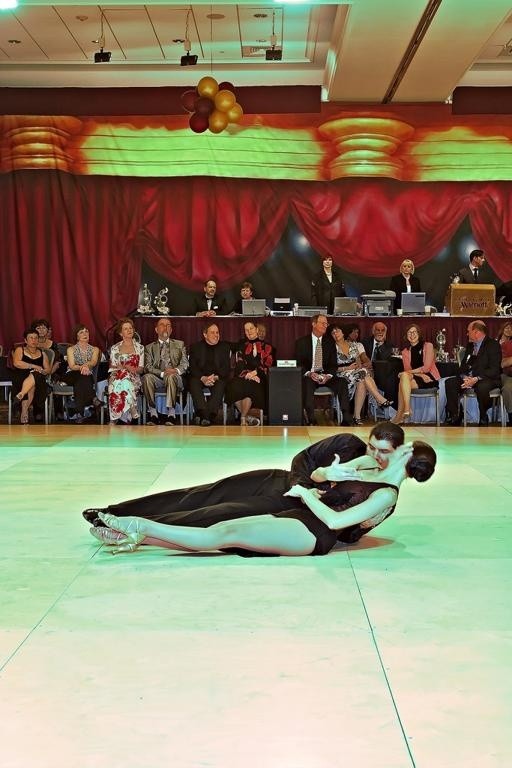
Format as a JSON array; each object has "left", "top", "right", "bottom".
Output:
[{"left": 447, "top": 283, "right": 496, "bottom": 316}]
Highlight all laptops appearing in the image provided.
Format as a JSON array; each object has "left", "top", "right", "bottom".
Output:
[
  {"left": 402, "top": 294, "right": 425, "bottom": 314},
  {"left": 334, "top": 297, "right": 357, "bottom": 314},
  {"left": 243, "top": 300, "right": 266, "bottom": 315}
]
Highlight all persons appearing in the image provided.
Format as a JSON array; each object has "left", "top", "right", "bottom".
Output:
[
  {"left": 187, "top": 325, "right": 232, "bottom": 427},
  {"left": 89, "top": 440, "right": 437, "bottom": 557},
  {"left": 457, "top": 250, "right": 491, "bottom": 284},
  {"left": 495, "top": 322, "right": 512, "bottom": 426},
  {"left": 32, "top": 319, "right": 61, "bottom": 423},
  {"left": 390, "top": 259, "right": 422, "bottom": 315},
  {"left": 392, "top": 326, "right": 440, "bottom": 427},
  {"left": 328, "top": 324, "right": 394, "bottom": 425},
  {"left": 343, "top": 324, "right": 360, "bottom": 342},
  {"left": 107, "top": 318, "right": 146, "bottom": 424},
  {"left": 311, "top": 254, "right": 347, "bottom": 314},
  {"left": 362, "top": 321, "right": 391, "bottom": 383},
  {"left": 294, "top": 314, "right": 354, "bottom": 428},
  {"left": 254, "top": 324, "right": 274, "bottom": 362},
  {"left": 82, "top": 421, "right": 404, "bottom": 529},
  {"left": 229, "top": 319, "right": 274, "bottom": 427},
  {"left": 444, "top": 321, "right": 502, "bottom": 429},
  {"left": 141, "top": 317, "right": 189, "bottom": 427},
  {"left": 195, "top": 279, "right": 227, "bottom": 318},
  {"left": 63, "top": 326, "right": 104, "bottom": 425},
  {"left": 233, "top": 282, "right": 257, "bottom": 315},
  {"left": 12, "top": 330, "right": 52, "bottom": 424}
]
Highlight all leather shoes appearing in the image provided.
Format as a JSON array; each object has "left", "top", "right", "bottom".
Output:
[
  {"left": 166, "top": 416, "right": 176, "bottom": 426},
  {"left": 147, "top": 415, "right": 158, "bottom": 425}
]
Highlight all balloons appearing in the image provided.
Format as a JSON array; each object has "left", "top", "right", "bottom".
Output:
[
  {"left": 218, "top": 81, "right": 236, "bottom": 94},
  {"left": 195, "top": 85, "right": 200, "bottom": 97},
  {"left": 195, "top": 98, "right": 214, "bottom": 115},
  {"left": 180, "top": 90, "right": 197, "bottom": 112},
  {"left": 190, "top": 112, "right": 208, "bottom": 133},
  {"left": 198, "top": 76, "right": 219, "bottom": 100},
  {"left": 208, "top": 110, "right": 228, "bottom": 133},
  {"left": 214, "top": 90, "right": 236, "bottom": 112},
  {"left": 227, "top": 103, "right": 244, "bottom": 123}
]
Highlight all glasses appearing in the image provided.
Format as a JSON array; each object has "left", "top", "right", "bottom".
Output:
[
  {"left": 408, "top": 330, "right": 419, "bottom": 334},
  {"left": 316, "top": 320, "right": 330, "bottom": 328}
]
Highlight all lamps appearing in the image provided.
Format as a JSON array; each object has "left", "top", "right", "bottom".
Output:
[
  {"left": 180, "top": 40, "right": 198, "bottom": 66},
  {"left": 266, "top": 33, "right": 281, "bottom": 63},
  {"left": 92, "top": 38, "right": 111, "bottom": 64}
]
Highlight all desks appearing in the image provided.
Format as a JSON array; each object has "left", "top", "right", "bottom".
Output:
[{"left": 130, "top": 312, "right": 511, "bottom": 375}]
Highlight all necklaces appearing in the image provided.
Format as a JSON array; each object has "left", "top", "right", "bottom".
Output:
[{"left": 27, "top": 348, "right": 36, "bottom": 357}]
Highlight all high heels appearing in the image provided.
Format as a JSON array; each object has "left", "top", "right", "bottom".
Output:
[
  {"left": 90, "top": 527, "right": 139, "bottom": 553},
  {"left": 402, "top": 409, "right": 414, "bottom": 418},
  {"left": 378, "top": 400, "right": 394, "bottom": 411},
  {"left": 99, "top": 511, "right": 146, "bottom": 545},
  {"left": 354, "top": 416, "right": 362, "bottom": 425},
  {"left": 389, "top": 417, "right": 403, "bottom": 425}
]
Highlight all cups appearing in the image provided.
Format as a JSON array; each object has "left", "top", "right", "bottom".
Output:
[
  {"left": 452, "top": 348, "right": 458, "bottom": 360},
  {"left": 394, "top": 351, "right": 399, "bottom": 356},
  {"left": 392, "top": 348, "right": 398, "bottom": 353},
  {"left": 397, "top": 309, "right": 402, "bottom": 317},
  {"left": 425, "top": 306, "right": 430, "bottom": 317}
]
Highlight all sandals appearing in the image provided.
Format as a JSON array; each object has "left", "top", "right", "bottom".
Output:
[
  {"left": 240, "top": 417, "right": 248, "bottom": 430},
  {"left": 247, "top": 416, "right": 260, "bottom": 427}
]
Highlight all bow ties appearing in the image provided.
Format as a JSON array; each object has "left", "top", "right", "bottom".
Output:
[{"left": 206, "top": 296, "right": 214, "bottom": 301}]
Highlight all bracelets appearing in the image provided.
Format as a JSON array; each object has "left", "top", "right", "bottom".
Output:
[{"left": 28, "top": 363, "right": 34, "bottom": 369}]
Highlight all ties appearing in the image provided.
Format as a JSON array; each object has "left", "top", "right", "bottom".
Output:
[{"left": 473, "top": 269, "right": 478, "bottom": 278}]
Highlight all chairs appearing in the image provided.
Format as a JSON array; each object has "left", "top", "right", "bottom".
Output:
[
  {"left": 186, "top": 389, "right": 228, "bottom": 426},
  {"left": 313, "top": 383, "right": 342, "bottom": 426},
  {"left": 52, "top": 341, "right": 76, "bottom": 424},
  {"left": 408, "top": 387, "right": 441, "bottom": 426},
  {"left": 100, "top": 386, "right": 142, "bottom": 425},
  {"left": 458, "top": 347, "right": 505, "bottom": 427},
  {"left": 143, "top": 390, "right": 183, "bottom": 425},
  {"left": 0, "top": 371, "right": 14, "bottom": 425}
]
